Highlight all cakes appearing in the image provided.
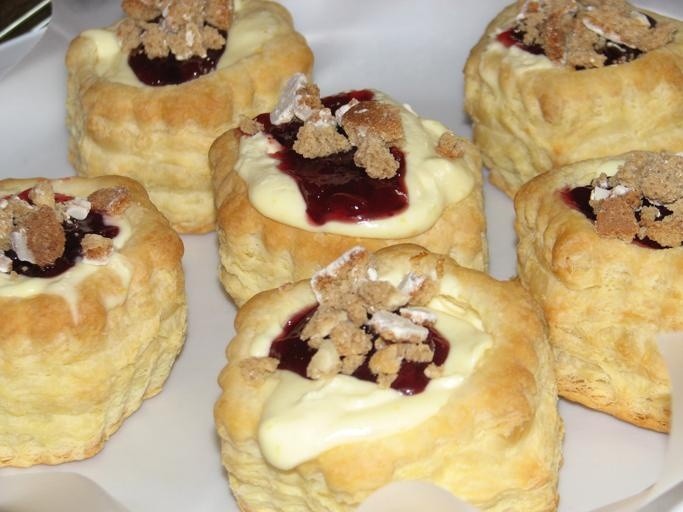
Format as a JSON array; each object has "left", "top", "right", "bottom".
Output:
[
  {"left": 212, "top": 243, "right": 566, "bottom": 510},
  {"left": 462, "top": 0, "right": 683, "bottom": 201},
  {"left": 0, "top": 176, "right": 188, "bottom": 468},
  {"left": 514, "top": 151, "right": 683, "bottom": 434},
  {"left": 65, "top": 1, "right": 313, "bottom": 235},
  {"left": 207, "top": 70, "right": 488, "bottom": 308}
]
